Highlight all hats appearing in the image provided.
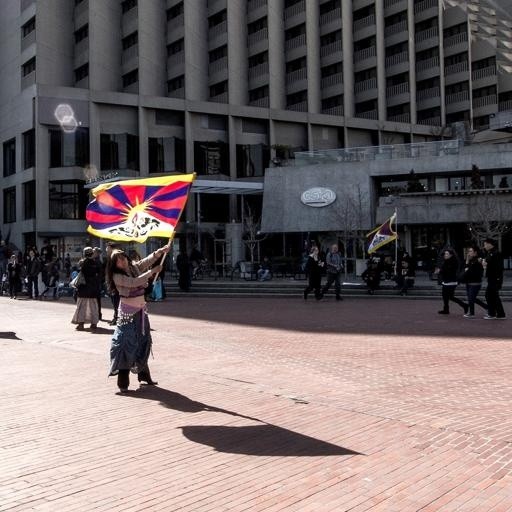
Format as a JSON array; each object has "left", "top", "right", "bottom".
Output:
[{"left": 83, "top": 246, "right": 93, "bottom": 254}]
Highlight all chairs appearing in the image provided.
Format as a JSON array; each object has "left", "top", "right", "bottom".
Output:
[{"left": 194, "top": 255, "right": 306, "bottom": 280}]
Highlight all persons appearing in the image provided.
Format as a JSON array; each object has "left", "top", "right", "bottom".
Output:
[
  {"left": 480, "top": 237, "right": 508, "bottom": 320},
  {"left": 462, "top": 246, "right": 495, "bottom": 318},
  {"left": 321, "top": 243, "right": 346, "bottom": 301},
  {"left": 438, "top": 250, "right": 470, "bottom": 316},
  {"left": 303, "top": 246, "right": 325, "bottom": 302},
  {"left": 360, "top": 249, "right": 416, "bottom": 296},
  {"left": 105, "top": 244, "right": 171, "bottom": 396},
  {"left": 426, "top": 239, "right": 440, "bottom": 280},
  {"left": 0, "top": 240, "right": 208, "bottom": 331}
]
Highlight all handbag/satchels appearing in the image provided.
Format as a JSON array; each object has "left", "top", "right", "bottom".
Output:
[{"left": 69, "top": 272, "right": 82, "bottom": 288}]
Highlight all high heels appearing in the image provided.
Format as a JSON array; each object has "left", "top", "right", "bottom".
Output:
[{"left": 138, "top": 378, "right": 158, "bottom": 385}]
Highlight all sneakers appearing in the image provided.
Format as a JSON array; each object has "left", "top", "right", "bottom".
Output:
[{"left": 438, "top": 304, "right": 507, "bottom": 320}]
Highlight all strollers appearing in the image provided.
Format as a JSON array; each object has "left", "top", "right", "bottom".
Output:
[{"left": 198, "top": 262, "right": 212, "bottom": 275}]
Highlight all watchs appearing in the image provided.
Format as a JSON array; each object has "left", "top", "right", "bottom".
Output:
[{"left": 256, "top": 256, "right": 272, "bottom": 282}]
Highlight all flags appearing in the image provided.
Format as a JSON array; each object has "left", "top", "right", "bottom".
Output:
[
  {"left": 85, "top": 173, "right": 193, "bottom": 244},
  {"left": 366, "top": 212, "right": 399, "bottom": 254}
]
